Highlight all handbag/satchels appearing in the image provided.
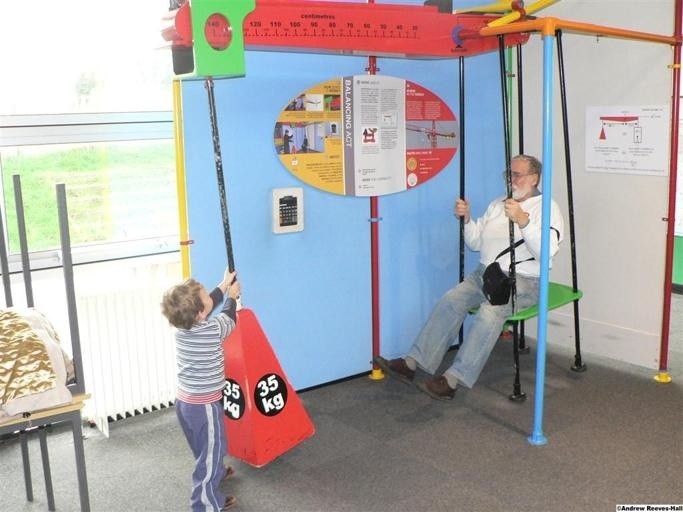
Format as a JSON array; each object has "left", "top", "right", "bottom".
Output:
[{"left": 481, "top": 226, "right": 561, "bottom": 307}]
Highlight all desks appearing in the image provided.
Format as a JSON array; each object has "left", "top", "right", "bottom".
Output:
[{"left": 0, "top": 174, "right": 94, "bottom": 512}]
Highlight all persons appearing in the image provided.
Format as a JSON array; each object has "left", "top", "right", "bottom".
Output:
[
  {"left": 300, "top": 134, "right": 308, "bottom": 153},
  {"left": 373, "top": 155, "right": 564, "bottom": 399},
  {"left": 330, "top": 124, "right": 337, "bottom": 133},
  {"left": 284, "top": 129, "right": 294, "bottom": 154},
  {"left": 159, "top": 267, "right": 240, "bottom": 511}
]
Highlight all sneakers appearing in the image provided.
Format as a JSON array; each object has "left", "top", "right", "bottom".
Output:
[
  {"left": 374, "top": 355, "right": 416, "bottom": 387},
  {"left": 413, "top": 372, "right": 457, "bottom": 404}
]
